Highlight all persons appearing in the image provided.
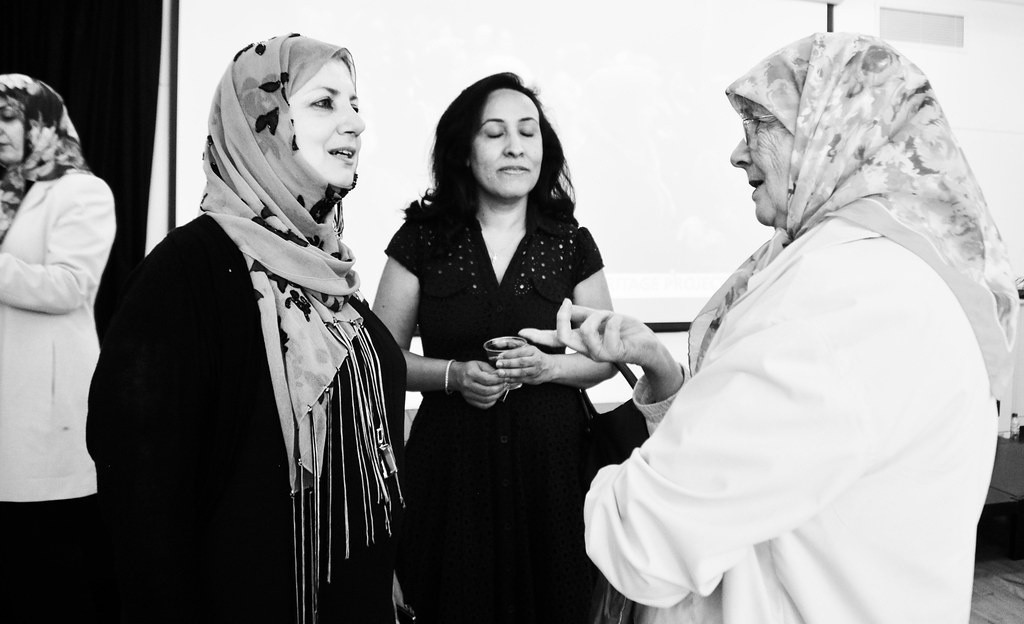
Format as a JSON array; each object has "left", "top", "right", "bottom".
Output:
[
  {"left": 88, "top": 34, "right": 409, "bottom": 624},
  {"left": 370, "top": 73, "right": 619, "bottom": 624},
  {"left": 518, "top": 34, "right": 1024, "bottom": 624},
  {"left": 0, "top": 73, "right": 115, "bottom": 624}
]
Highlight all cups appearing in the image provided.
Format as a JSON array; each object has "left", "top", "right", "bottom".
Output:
[{"left": 483, "top": 337, "right": 528, "bottom": 390}]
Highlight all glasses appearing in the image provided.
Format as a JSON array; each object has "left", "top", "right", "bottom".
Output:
[{"left": 741, "top": 114, "right": 779, "bottom": 146}]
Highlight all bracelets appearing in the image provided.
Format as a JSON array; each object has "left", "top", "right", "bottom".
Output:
[{"left": 445, "top": 360, "right": 456, "bottom": 395}]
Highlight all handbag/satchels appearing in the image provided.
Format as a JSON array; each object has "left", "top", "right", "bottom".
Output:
[{"left": 579, "top": 356, "right": 649, "bottom": 465}]
